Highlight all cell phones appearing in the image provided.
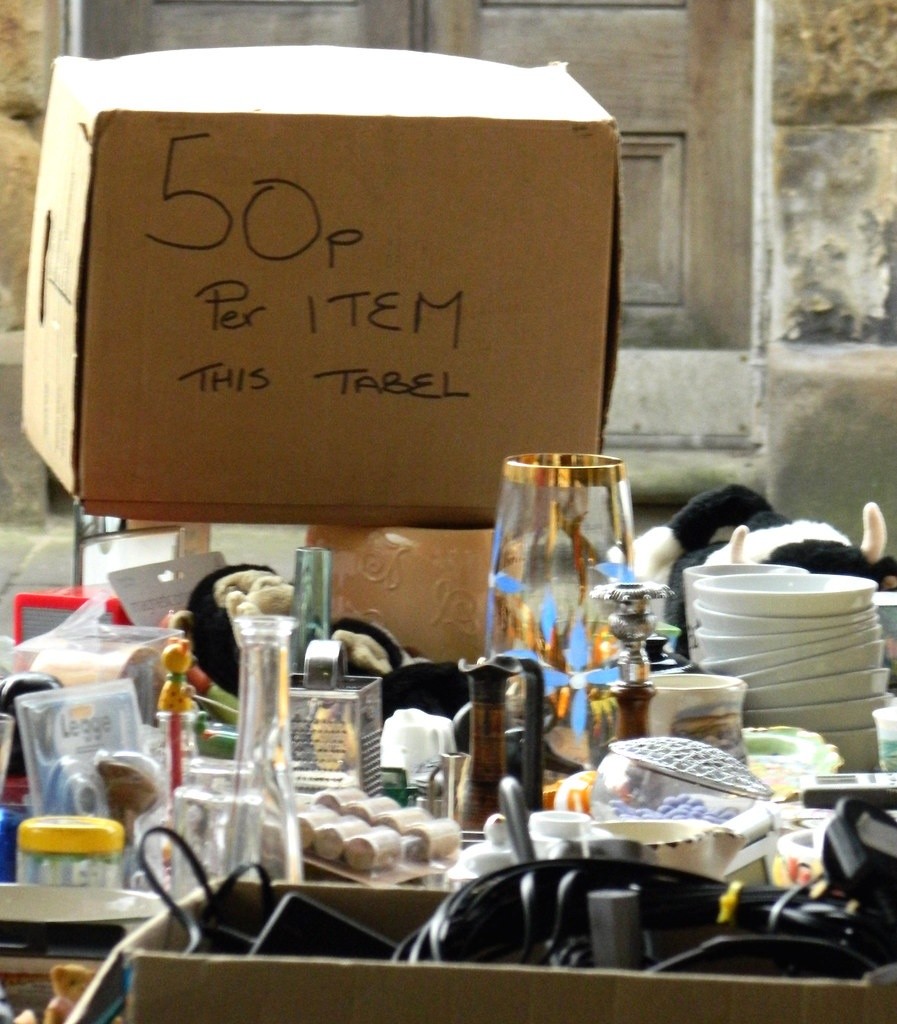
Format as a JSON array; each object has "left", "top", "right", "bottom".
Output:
[{"left": 248, "top": 893, "right": 411, "bottom": 961}]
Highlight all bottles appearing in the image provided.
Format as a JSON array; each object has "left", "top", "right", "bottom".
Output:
[
  {"left": 170, "top": 757, "right": 262, "bottom": 901},
  {"left": 223, "top": 614, "right": 306, "bottom": 885},
  {"left": 282, "top": 544, "right": 333, "bottom": 688},
  {"left": 15, "top": 815, "right": 125, "bottom": 889}
]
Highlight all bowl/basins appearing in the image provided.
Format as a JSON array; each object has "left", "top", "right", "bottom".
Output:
[
  {"left": 691, "top": 573, "right": 895, "bottom": 774},
  {"left": 0, "top": 883, "right": 164, "bottom": 933}
]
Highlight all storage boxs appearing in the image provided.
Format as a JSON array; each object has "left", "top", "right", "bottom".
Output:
[
  {"left": 26, "top": 56, "right": 628, "bottom": 524},
  {"left": 120, "top": 872, "right": 897, "bottom": 1023}
]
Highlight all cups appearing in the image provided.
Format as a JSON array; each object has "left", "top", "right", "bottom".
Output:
[
  {"left": 682, "top": 563, "right": 810, "bottom": 663},
  {"left": 528, "top": 810, "right": 592, "bottom": 861},
  {"left": 872, "top": 706, "right": 897, "bottom": 772},
  {"left": 648, "top": 674, "right": 749, "bottom": 767},
  {"left": 482, "top": 452, "right": 639, "bottom": 774}
]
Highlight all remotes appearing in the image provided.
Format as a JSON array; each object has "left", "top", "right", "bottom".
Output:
[{"left": 802, "top": 772, "right": 897, "bottom": 813}]
